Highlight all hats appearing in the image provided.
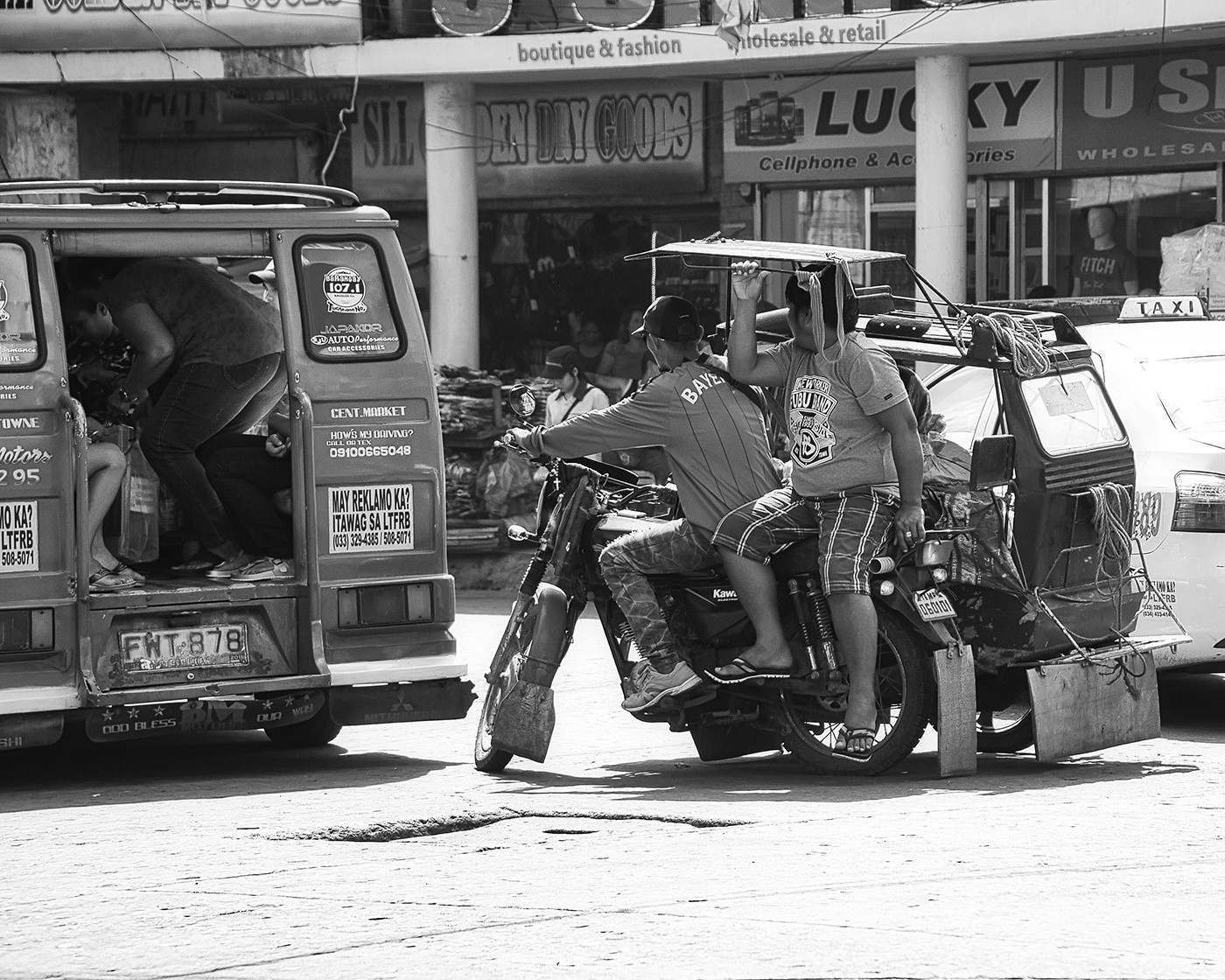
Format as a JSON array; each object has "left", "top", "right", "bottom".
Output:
[
  {"left": 541, "top": 345, "right": 582, "bottom": 379},
  {"left": 631, "top": 295, "right": 701, "bottom": 342}
]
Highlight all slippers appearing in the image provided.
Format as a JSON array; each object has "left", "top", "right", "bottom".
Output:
[
  {"left": 74, "top": 563, "right": 146, "bottom": 592},
  {"left": 704, "top": 657, "right": 791, "bottom": 686},
  {"left": 831, "top": 717, "right": 880, "bottom": 763}
]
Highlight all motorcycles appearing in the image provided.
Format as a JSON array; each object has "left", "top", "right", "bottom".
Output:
[{"left": 474, "top": 231, "right": 1188, "bottom": 777}]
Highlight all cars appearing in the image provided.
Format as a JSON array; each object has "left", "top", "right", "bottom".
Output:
[{"left": 922, "top": 322, "right": 1225, "bottom": 712}]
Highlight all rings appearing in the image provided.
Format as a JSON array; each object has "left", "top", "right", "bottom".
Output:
[{"left": 905, "top": 531, "right": 912, "bottom": 538}]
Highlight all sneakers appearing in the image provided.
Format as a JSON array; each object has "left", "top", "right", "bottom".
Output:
[{"left": 621, "top": 661, "right": 704, "bottom": 713}]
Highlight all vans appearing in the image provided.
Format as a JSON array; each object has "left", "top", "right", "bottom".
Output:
[{"left": 0, "top": 178, "right": 478, "bottom": 754}]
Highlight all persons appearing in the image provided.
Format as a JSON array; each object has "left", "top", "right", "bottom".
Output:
[
  {"left": 1070, "top": 206, "right": 1139, "bottom": 296},
  {"left": 704, "top": 259, "right": 924, "bottom": 762},
  {"left": 545, "top": 307, "right": 671, "bottom": 484},
  {"left": 507, "top": 295, "right": 783, "bottom": 710},
  {"left": 56, "top": 254, "right": 357, "bottom": 592}
]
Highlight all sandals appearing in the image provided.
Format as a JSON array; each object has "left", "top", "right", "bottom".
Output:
[
  {"left": 205, "top": 555, "right": 250, "bottom": 578},
  {"left": 230, "top": 556, "right": 295, "bottom": 581}
]
